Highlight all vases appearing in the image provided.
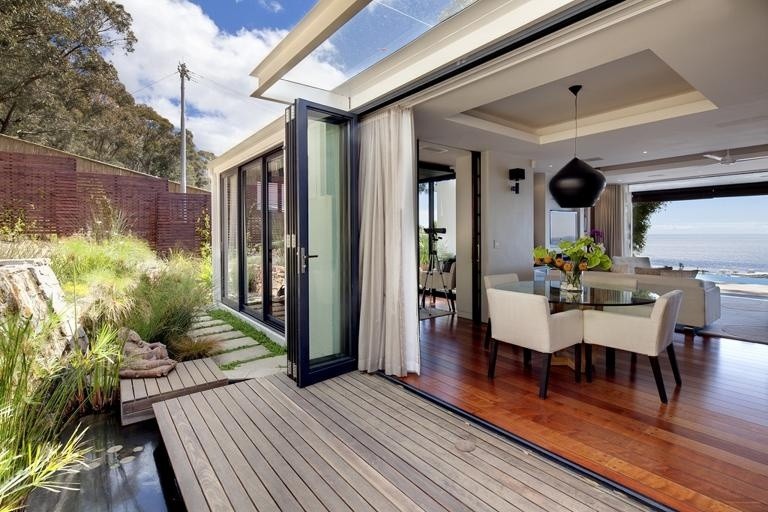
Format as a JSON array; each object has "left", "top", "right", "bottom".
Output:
[{"left": 557, "top": 270, "right": 585, "bottom": 304}]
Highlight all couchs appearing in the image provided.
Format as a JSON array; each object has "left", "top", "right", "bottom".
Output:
[
  {"left": 609, "top": 253, "right": 652, "bottom": 274},
  {"left": 550, "top": 266, "right": 723, "bottom": 330},
  {"left": 419, "top": 264, "right": 461, "bottom": 318}
]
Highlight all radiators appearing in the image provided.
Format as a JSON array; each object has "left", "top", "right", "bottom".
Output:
[{"left": 494, "top": 278, "right": 660, "bottom": 375}]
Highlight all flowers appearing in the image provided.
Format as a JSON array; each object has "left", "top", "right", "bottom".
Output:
[{"left": 530, "top": 234, "right": 614, "bottom": 277}]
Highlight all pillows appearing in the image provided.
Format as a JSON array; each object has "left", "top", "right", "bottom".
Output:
[
  {"left": 633, "top": 265, "right": 672, "bottom": 278},
  {"left": 658, "top": 268, "right": 700, "bottom": 282}
]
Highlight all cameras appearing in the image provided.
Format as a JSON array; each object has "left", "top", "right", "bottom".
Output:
[{"left": 424, "top": 227, "right": 446, "bottom": 251}]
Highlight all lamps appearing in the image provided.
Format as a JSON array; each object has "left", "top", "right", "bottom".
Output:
[
  {"left": 508, "top": 167, "right": 526, "bottom": 194},
  {"left": 548, "top": 83, "right": 607, "bottom": 209}
]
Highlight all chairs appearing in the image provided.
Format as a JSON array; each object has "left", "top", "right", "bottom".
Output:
[
  {"left": 481, "top": 271, "right": 519, "bottom": 348},
  {"left": 485, "top": 287, "right": 585, "bottom": 400},
  {"left": 582, "top": 289, "right": 683, "bottom": 406}
]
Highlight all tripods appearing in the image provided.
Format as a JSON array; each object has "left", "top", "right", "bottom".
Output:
[{"left": 419, "top": 252, "right": 451, "bottom": 316}]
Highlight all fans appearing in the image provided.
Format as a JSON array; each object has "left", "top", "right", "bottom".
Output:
[{"left": 701, "top": 147, "right": 767, "bottom": 169}]
